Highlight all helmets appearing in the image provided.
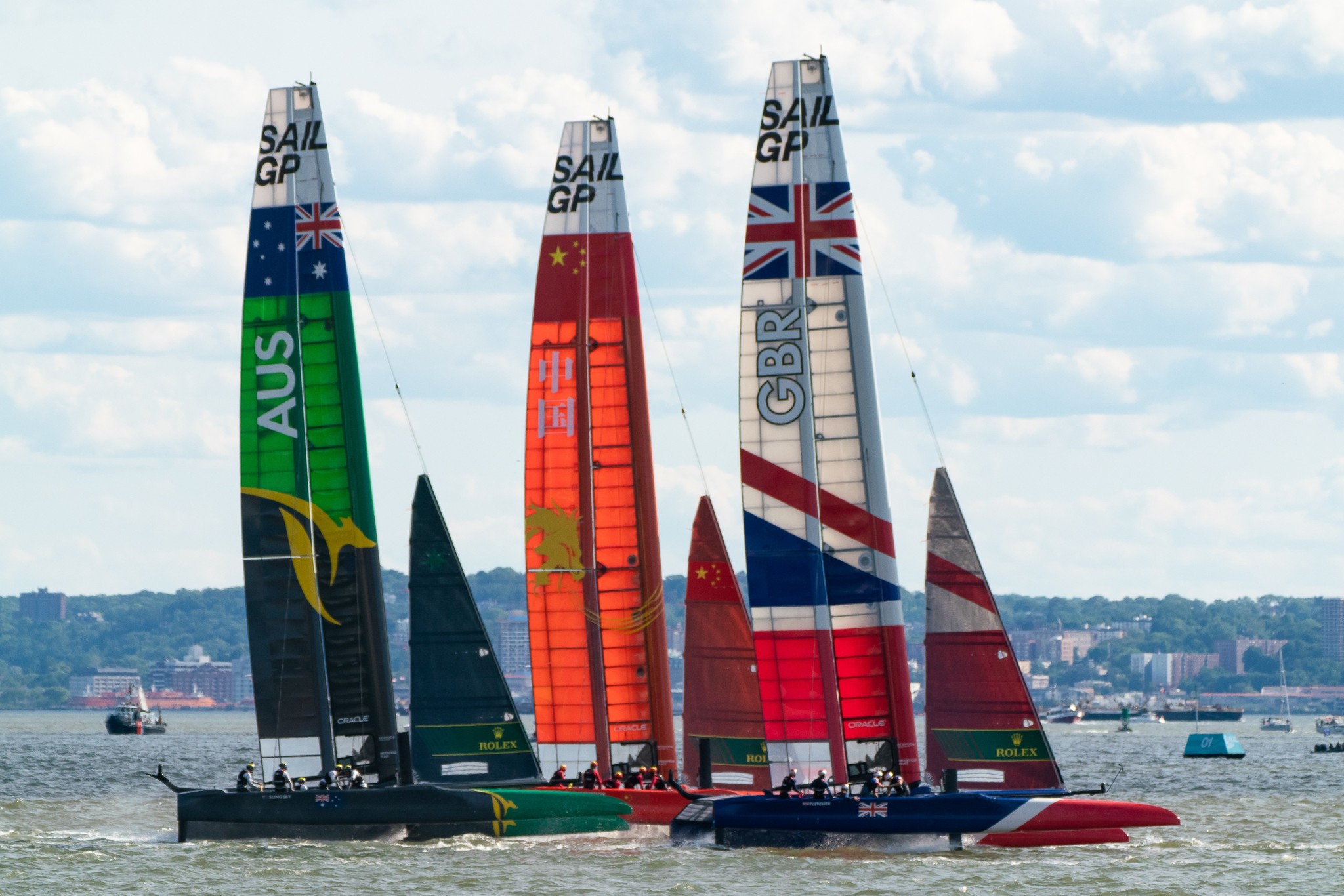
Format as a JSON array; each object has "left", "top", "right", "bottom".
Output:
[
  {"left": 639, "top": 767, "right": 647, "bottom": 773},
  {"left": 790, "top": 769, "right": 797, "bottom": 775},
  {"left": 246, "top": 764, "right": 255, "bottom": 772},
  {"left": 842, "top": 784, "right": 849, "bottom": 792},
  {"left": 560, "top": 764, "right": 567, "bottom": 771},
  {"left": 335, "top": 764, "right": 343, "bottom": 771},
  {"left": 344, "top": 766, "right": 351, "bottom": 771},
  {"left": 591, "top": 762, "right": 597, "bottom": 767},
  {"left": 298, "top": 778, "right": 305, "bottom": 784},
  {"left": 278, "top": 763, "right": 287, "bottom": 770},
  {"left": 818, "top": 769, "right": 827, "bottom": 777},
  {"left": 874, "top": 771, "right": 883, "bottom": 777},
  {"left": 884, "top": 772, "right": 894, "bottom": 781},
  {"left": 615, "top": 771, "right": 623, "bottom": 778},
  {"left": 648, "top": 767, "right": 657, "bottom": 773}
]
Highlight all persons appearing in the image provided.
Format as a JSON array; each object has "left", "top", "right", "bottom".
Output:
[
  {"left": 340, "top": 765, "right": 367, "bottom": 789},
  {"left": 272, "top": 762, "right": 294, "bottom": 791},
  {"left": 808, "top": 769, "right": 837, "bottom": 799},
  {"left": 624, "top": 767, "right": 647, "bottom": 790},
  {"left": 549, "top": 765, "right": 568, "bottom": 788},
  {"left": 294, "top": 778, "right": 308, "bottom": 791},
  {"left": 236, "top": 762, "right": 263, "bottom": 792},
  {"left": 645, "top": 767, "right": 666, "bottom": 790},
  {"left": 583, "top": 761, "right": 602, "bottom": 790},
  {"left": 884, "top": 771, "right": 910, "bottom": 797},
  {"left": 860, "top": 770, "right": 885, "bottom": 797},
  {"left": 835, "top": 784, "right": 860, "bottom": 801},
  {"left": 602, "top": 772, "right": 623, "bottom": 789},
  {"left": 779, "top": 768, "right": 803, "bottom": 799},
  {"left": 319, "top": 764, "right": 343, "bottom": 790}
]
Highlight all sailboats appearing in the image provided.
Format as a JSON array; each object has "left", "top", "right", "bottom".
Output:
[
  {"left": 146, "top": 37, "right": 1186, "bottom": 863},
  {"left": 1259, "top": 645, "right": 1293, "bottom": 733}
]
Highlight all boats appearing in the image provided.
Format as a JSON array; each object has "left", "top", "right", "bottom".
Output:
[
  {"left": 1183, "top": 686, "right": 1246, "bottom": 760},
  {"left": 1148, "top": 683, "right": 1245, "bottom": 723},
  {"left": 104, "top": 682, "right": 167, "bottom": 735},
  {"left": 1315, "top": 715, "right": 1344, "bottom": 735}
]
